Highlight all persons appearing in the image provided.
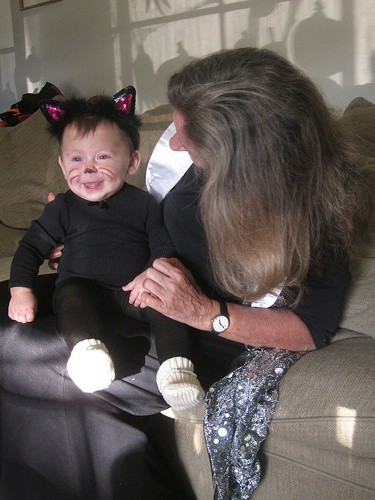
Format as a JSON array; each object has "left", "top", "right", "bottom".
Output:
[
  {"left": 8, "top": 85, "right": 209, "bottom": 423},
  {"left": 0, "top": 46, "right": 363, "bottom": 499}
]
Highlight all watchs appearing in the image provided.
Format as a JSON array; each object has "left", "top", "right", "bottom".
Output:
[{"left": 211, "top": 300, "right": 231, "bottom": 335}]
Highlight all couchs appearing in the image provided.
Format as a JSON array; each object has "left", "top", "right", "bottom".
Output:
[{"left": 0, "top": 96, "right": 375, "bottom": 500}]
